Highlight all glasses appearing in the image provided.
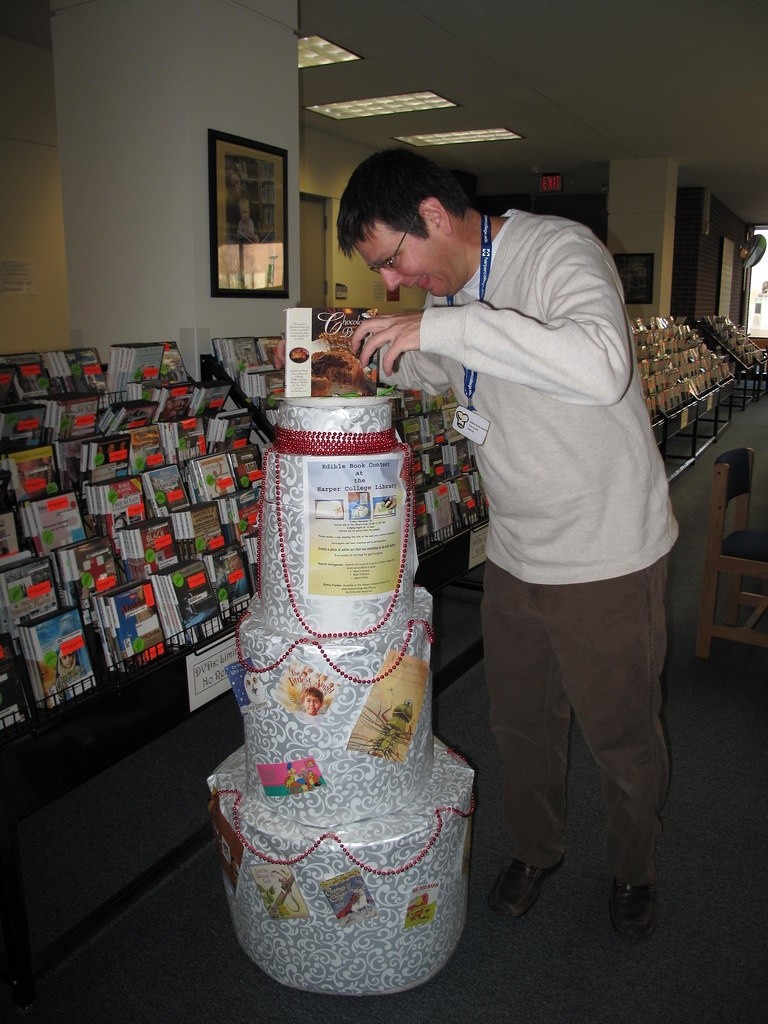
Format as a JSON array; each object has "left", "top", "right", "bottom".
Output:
[{"left": 368, "top": 211, "right": 416, "bottom": 273}]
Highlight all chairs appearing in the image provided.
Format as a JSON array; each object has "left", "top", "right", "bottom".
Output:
[{"left": 696, "top": 446, "right": 768, "bottom": 659}]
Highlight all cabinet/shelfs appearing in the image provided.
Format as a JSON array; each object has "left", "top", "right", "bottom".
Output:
[{"left": 1, "top": 317, "right": 768, "bottom": 1014}]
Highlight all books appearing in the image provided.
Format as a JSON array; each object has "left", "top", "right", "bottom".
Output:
[
  {"left": 0, "top": 342, "right": 264, "bottom": 730},
  {"left": 225, "top": 156, "right": 248, "bottom": 179},
  {"left": 702, "top": 314, "right": 763, "bottom": 365},
  {"left": 631, "top": 315, "right": 729, "bottom": 418},
  {"left": 284, "top": 306, "right": 380, "bottom": 396},
  {"left": 211, "top": 334, "right": 286, "bottom": 416},
  {"left": 258, "top": 162, "right": 274, "bottom": 226},
  {"left": 376, "top": 384, "right": 488, "bottom": 549}
]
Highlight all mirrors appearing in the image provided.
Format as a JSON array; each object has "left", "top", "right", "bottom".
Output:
[{"left": 738, "top": 234, "right": 767, "bottom": 268}]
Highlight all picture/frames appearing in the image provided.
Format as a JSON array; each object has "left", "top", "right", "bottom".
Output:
[{"left": 207, "top": 127, "right": 292, "bottom": 300}]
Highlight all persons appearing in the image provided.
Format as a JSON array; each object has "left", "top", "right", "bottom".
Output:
[
  {"left": 273, "top": 148, "right": 680, "bottom": 939},
  {"left": 225, "top": 169, "right": 261, "bottom": 244},
  {"left": 302, "top": 688, "right": 324, "bottom": 716}
]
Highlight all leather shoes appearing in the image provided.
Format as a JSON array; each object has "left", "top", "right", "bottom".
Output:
[
  {"left": 487, "top": 859, "right": 544, "bottom": 920},
  {"left": 609, "top": 879, "right": 657, "bottom": 939}
]
